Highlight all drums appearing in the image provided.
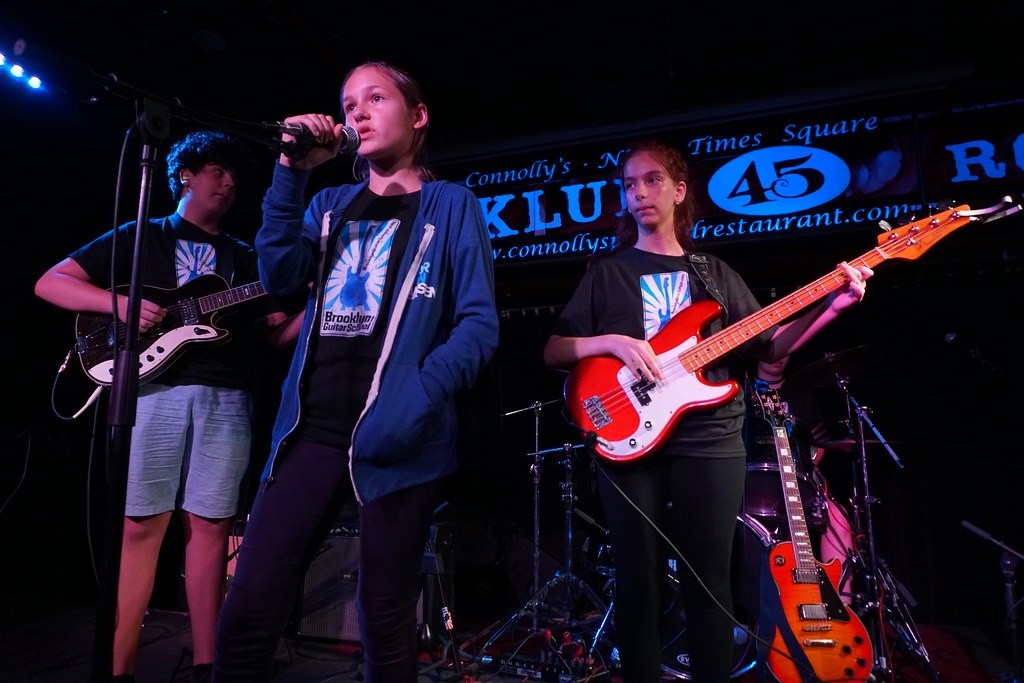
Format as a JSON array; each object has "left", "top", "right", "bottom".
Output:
[
  {"left": 656, "top": 512, "right": 782, "bottom": 681},
  {"left": 740, "top": 407, "right": 819, "bottom": 519}
]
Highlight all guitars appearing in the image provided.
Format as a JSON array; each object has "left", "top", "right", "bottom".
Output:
[
  {"left": 561, "top": 197, "right": 973, "bottom": 460},
  {"left": 740, "top": 383, "right": 874, "bottom": 683},
  {"left": 73, "top": 273, "right": 269, "bottom": 390}
]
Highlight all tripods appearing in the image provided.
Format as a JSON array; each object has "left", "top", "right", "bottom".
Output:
[
  {"left": 454, "top": 394, "right": 618, "bottom": 667},
  {"left": 823, "top": 359, "right": 946, "bottom": 683}
]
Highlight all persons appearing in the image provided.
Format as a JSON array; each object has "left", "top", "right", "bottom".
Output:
[
  {"left": 543, "top": 145, "right": 874, "bottom": 683},
  {"left": 34, "top": 131, "right": 306, "bottom": 683},
  {"left": 210, "top": 61, "right": 501, "bottom": 683}
]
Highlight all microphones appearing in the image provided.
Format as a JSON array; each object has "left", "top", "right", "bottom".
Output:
[
  {"left": 341, "top": 570, "right": 359, "bottom": 584},
  {"left": 945, "top": 333, "right": 985, "bottom": 365},
  {"left": 262, "top": 120, "right": 362, "bottom": 156}
]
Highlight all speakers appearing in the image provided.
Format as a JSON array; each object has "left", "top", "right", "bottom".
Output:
[{"left": 294, "top": 520, "right": 454, "bottom": 652}]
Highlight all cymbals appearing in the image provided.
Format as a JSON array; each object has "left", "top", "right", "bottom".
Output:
[
  {"left": 816, "top": 434, "right": 899, "bottom": 447},
  {"left": 784, "top": 342, "right": 879, "bottom": 385}
]
